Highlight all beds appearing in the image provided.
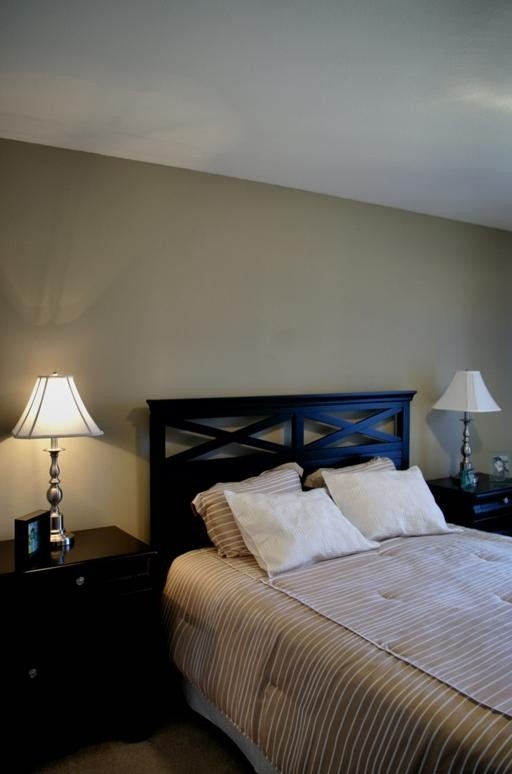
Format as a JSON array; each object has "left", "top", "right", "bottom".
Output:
[{"left": 147, "top": 387, "right": 511, "bottom": 774}]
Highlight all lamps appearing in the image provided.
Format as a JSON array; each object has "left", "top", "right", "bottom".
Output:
[
  {"left": 12, "top": 372, "right": 105, "bottom": 548},
  {"left": 433, "top": 366, "right": 504, "bottom": 489}
]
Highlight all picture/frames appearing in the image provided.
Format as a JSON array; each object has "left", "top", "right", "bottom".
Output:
[{"left": 13, "top": 509, "right": 50, "bottom": 565}]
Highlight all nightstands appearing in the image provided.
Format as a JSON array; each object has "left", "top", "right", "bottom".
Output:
[
  {"left": 1, "top": 524, "right": 174, "bottom": 774},
  {"left": 426, "top": 472, "right": 512, "bottom": 537}
]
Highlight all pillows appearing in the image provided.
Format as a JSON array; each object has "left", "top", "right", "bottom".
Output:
[
  {"left": 306, "top": 455, "right": 396, "bottom": 488},
  {"left": 321, "top": 466, "right": 449, "bottom": 541},
  {"left": 189, "top": 462, "right": 305, "bottom": 558},
  {"left": 221, "top": 487, "right": 383, "bottom": 575}
]
